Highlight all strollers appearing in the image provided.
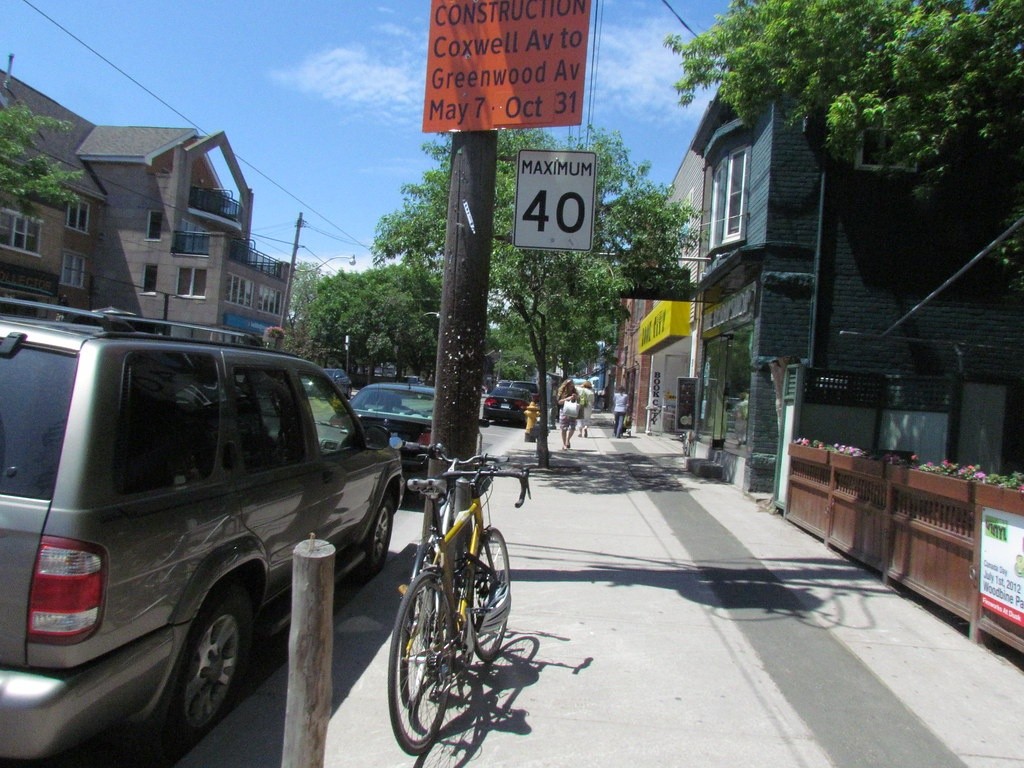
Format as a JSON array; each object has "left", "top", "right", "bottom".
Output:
[{"left": 620, "top": 404, "right": 632, "bottom": 438}]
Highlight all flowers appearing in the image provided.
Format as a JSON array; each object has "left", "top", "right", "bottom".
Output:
[
  {"left": 266, "top": 326, "right": 284, "bottom": 339},
  {"left": 787, "top": 435, "right": 829, "bottom": 452},
  {"left": 828, "top": 441, "right": 882, "bottom": 462},
  {"left": 974, "top": 469, "right": 1024, "bottom": 497},
  {"left": 884, "top": 452, "right": 981, "bottom": 484}
]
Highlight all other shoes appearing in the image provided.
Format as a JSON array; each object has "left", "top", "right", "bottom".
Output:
[
  {"left": 584, "top": 428, "right": 587, "bottom": 437},
  {"left": 565, "top": 443, "right": 570, "bottom": 448},
  {"left": 578, "top": 433, "right": 582, "bottom": 437}
]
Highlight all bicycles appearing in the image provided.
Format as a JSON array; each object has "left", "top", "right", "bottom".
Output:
[
  {"left": 387, "top": 469, "right": 531, "bottom": 757},
  {"left": 399, "top": 441, "right": 508, "bottom": 709}
]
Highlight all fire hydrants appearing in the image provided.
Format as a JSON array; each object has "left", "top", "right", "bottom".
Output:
[{"left": 523, "top": 401, "right": 539, "bottom": 433}]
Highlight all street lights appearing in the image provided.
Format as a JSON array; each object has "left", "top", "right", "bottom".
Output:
[{"left": 274, "top": 255, "right": 356, "bottom": 352}]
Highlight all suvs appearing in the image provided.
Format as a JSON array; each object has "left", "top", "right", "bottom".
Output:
[{"left": 1, "top": 296, "right": 403, "bottom": 763}]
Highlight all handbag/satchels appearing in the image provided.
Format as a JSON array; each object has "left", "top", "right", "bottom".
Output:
[
  {"left": 563, "top": 395, "right": 580, "bottom": 418},
  {"left": 580, "top": 388, "right": 588, "bottom": 406},
  {"left": 623, "top": 413, "right": 627, "bottom": 428}
]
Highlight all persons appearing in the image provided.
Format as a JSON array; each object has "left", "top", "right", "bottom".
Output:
[
  {"left": 576, "top": 380, "right": 594, "bottom": 438},
  {"left": 613, "top": 386, "right": 629, "bottom": 438},
  {"left": 557, "top": 379, "right": 580, "bottom": 451}
]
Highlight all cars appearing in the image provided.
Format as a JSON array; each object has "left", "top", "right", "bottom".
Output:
[
  {"left": 483, "top": 386, "right": 532, "bottom": 426},
  {"left": 497, "top": 379, "right": 540, "bottom": 403},
  {"left": 348, "top": 383, "right": 434, "bottom": 500}
]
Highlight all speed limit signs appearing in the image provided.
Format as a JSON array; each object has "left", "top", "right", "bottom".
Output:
[{"left": 512, "top": 149, "right": 597, "bottom": 252}]
